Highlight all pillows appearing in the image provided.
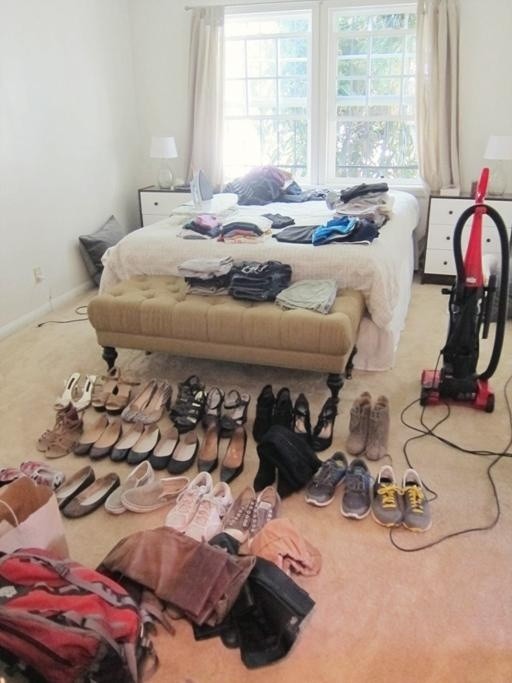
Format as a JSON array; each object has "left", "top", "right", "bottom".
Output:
[{"left": 79, "top": 215, "right": 127, "bottom": 286}]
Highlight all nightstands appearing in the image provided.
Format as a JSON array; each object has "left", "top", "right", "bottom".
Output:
[
  {"left": 138, "top": 184, "right": 193, "bottom": 228},
  {"left": 420, "top": 191, "right": 512, "bottom": 286}
]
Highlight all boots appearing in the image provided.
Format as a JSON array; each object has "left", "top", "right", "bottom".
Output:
[
  {"left": 94, "top": 525, "right": 315, "bottom": 671},
  {"left": 363, "top": 393, "right": 393, "bottom": 462},
  {"left": 345, "top": 390, "right": 373, "bottom": 457},
  {"left": 253, "top": 423, "right": 323, "bottom": 500}
]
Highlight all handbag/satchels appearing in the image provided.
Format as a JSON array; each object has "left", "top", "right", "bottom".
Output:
[{"left": 0, "top": 475, "right": 71, "bottom": 561}]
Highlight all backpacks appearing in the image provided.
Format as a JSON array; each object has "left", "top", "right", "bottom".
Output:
[{"left": 0, "top": 548, "right": 159, "bottom": 683}]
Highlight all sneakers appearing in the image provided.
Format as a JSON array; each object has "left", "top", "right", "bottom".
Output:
[
  {"left": 106, "top": 469, "right": 231, "bottom": 542},
  {"left": 251, "top": 384, "right": 274, "bottom": 441},
  {"left": 220, "top": 484, "right": 281, "bottom": 545},
  {"left": 338, "top": 457, "right": 375, "bottom": 522},
  {"left": 400, "top": 467, "right": 434, "bottom": 536},
  {"left": 301, "top": 448, "right": 350, "bottom": 508},
  {"left": 369, "top": 464, "right": 402, "bottom": 528},
  {"left": 18, "top": 458, "right": 63, "bottom": 488},
  {"left": 274, "top": 385, "right": 292, "bottom": 430}
]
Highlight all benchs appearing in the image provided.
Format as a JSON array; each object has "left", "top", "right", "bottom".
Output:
[{"left": 87, "top": 274, "right": 367, "bottom": 404}]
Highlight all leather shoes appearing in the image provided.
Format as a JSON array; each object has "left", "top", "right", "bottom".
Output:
[
  {"left": 219, "top": 426, "right": 248, "bottom": 482},
  {"left": 166, "top": 430, "right": 200, "bottom": 474},
  {"left": 43, "top": 464, "right": 96, "bottom": 511},
  {"left": 307, "top": 396, "right": 341, "bottom": 450},
  {"left": 147, "top": 426, "right": 181, "bottom": 471},
  {"left": 108, "top": 420, "right": 143, "bottom": 461},
  {"left": 62, "top": 471, "right": 121, "bottom": 519},
  {"left": 89, "top": 416, "right": 124, "bottom": 460},
  {"left": 73, "top": 411, "right": 111, "bottom": 456},
  {"left": 292, "top": 391, "right": 314, "bottom": 448},
  {"left": 126, "top": 420, "right": 161, "bottom": 465},
  {"left": 197, "top": 421, "right": 222, "bottom": 474}
]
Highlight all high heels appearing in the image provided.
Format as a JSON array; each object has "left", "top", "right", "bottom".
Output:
[
  {"left": 133, "top": 379, "right": 175, "bottom": 426},
  {"left": 221, "top": 388, "right": 251, "bottom": 430},
  {"left": 120, "top": 377, "right": 159, "bottom": 423},
  {"left": 53, "top": 370, "right": 81, "bottom": 411},
  {"left": 201, "top": 384, "right": 225, "bottom": 431},
  {"left": 72, "top": 372, "right": 98, "bottom": 413}
]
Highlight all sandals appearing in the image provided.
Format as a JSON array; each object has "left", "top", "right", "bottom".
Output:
[
  {"left": 173, "top": 383, "right": 208, "bottom": 433},
  {"left": 45, "top": 407, "right": 86, "bottom": 460},
  {"left": 91, "top": 366, "right": 121, "bottom": 414},
  {"left": 167, "top": 373, "right": 206, "bottom": 419},
  {"left": 35, "top": 401, "right": 74, "bottom": 452},
  {"left": 105, "top": 374, "right": 141, "bottom": 416}
]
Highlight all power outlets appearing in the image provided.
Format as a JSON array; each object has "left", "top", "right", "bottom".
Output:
[{"left": 34, "top": 268, "right": 42, "bottom": 283}]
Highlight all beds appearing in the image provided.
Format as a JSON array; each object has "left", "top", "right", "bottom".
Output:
[{"left": 97, "top": 190, "right": 420, "bottom": 372}]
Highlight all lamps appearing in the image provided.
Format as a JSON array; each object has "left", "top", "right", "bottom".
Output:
[
  {"left": 150, "top": 136, "right": 178, "bottom": 188},
  {"left": 483, "top": 134, "right": 512, "bottom": 195}
]
